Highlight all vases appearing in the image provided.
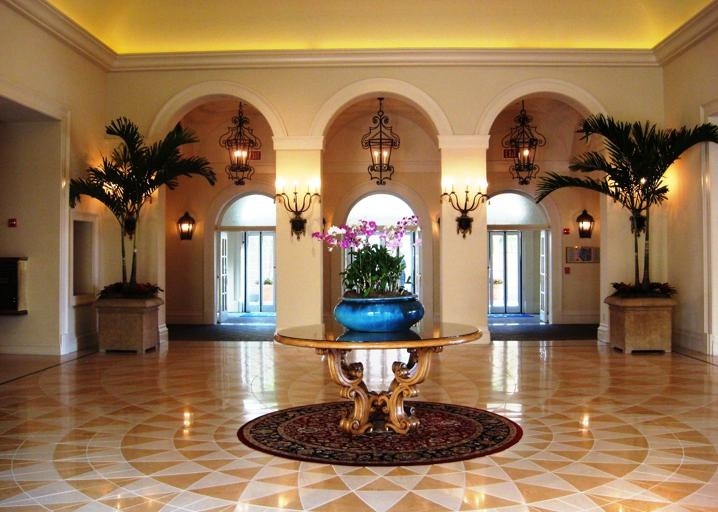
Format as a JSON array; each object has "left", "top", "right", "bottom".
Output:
[{"left": 334, "top": 297, "right": 426, "bottom": 332}]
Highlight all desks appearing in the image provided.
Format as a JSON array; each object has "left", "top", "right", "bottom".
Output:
[{"left": 273, "top": 321, "right": 483, "bottom": 436}]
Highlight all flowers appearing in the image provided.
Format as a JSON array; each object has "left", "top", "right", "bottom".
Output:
[{"left": 310, "top": 213, "right": 424, "bottom": 296}]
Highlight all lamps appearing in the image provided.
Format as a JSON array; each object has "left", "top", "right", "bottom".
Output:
[
  {"left": 176, "top": 210, "right": 195, "bottom": 240},
  {"left": 271, "top": 178, "right": 322, "bottom": 242},
  {"left": 219, "top": 101, "right": 262, "bottom": 186},
  {"left": 438, "top": 172, "right": 490, "bottom": 239},
  {"left": 359, "top": 97, "right": 401, "bottom": 187},
  {"left": 575, "top": 209, "right": 595, "bottom": 240},
  {"left": 501, "top": 96, "right": 546, "bottom": 186}
]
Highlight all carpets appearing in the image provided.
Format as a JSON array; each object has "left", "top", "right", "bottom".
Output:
[{"left": 236, "top": 399, "right": 524, "bottom": 467}]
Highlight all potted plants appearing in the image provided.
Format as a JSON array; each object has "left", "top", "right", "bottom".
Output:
[
  {"left": 531, "top": 111, "right": 718, "bottom": 356},
  {"left": 68, "top": 115, "right": 220, "bottom": 357}
]
